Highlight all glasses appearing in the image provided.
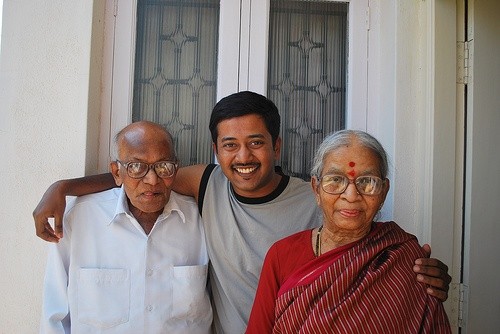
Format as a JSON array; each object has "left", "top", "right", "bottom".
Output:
[
  {"left": 117, "top": 159, "right": 178, "bottom": 179},
  {"left": 317, "top": 172, "right": 387, "bottom": 195}
]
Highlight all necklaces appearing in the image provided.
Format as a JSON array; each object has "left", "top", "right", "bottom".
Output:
[{"left": 316, "top": 225, "right": 324, "bottom": 258}]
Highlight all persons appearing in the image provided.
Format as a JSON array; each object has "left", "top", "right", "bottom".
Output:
[
  {"left": 34, "top": 91, "right": 452, "bottom": 334},
  {"left": 246, "top": 130, "right": 452, "bottom": 334},
  {"left": 39, "top": 120, "right": 214, "bottom": 334}
]
